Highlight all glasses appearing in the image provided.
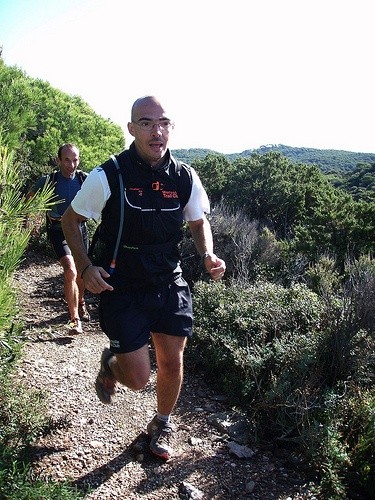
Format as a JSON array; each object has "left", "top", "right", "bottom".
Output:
[{"left": 132, "top": 120, "right": 171, "bottom": 130}]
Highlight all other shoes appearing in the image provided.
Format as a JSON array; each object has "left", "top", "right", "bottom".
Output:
[
  {"left": 69, "top": 318, "right": 83, "bottom": 335},
  {"left": 79, "top": 304, "right": 91, "bottom": 321}
]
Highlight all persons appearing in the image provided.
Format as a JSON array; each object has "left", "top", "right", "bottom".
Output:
[
  {"left": 61, "top": 96, "right": 227, "bottom": 461},
  {"left": 23, "top": 143, "right": 91, "bottom": 335}
]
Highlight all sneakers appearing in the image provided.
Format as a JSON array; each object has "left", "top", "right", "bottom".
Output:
[
  {"left": 96, "top": 348, "right": 119, "bottom": 403},
  {"left": 147, "top": 416, "right": 172, "bottom": 459}
]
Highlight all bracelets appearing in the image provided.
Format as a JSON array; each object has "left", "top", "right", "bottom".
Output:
[
  {"left": 79, "top": 262, "right": 92, "bottom": 279},
  {"left": 200, "top": 251, "right": 213, "bottom": 262}
]
